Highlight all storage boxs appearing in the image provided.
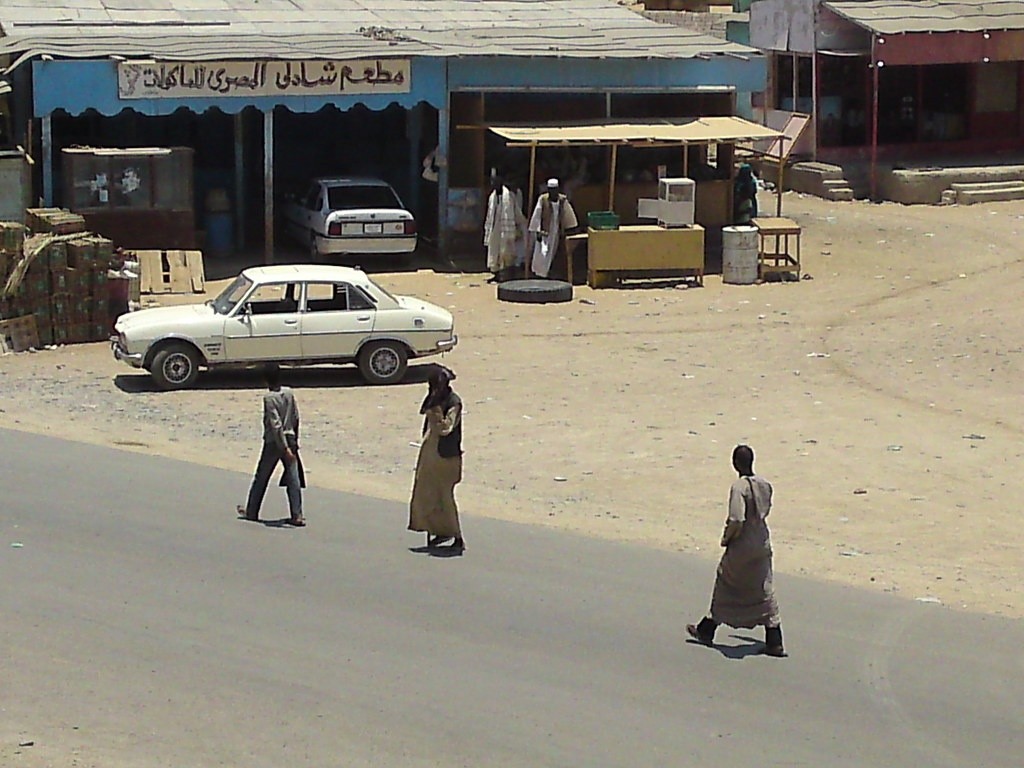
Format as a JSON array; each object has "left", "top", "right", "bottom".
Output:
[
  {"left": 0, "top": 207, "right": 129, "bottom": 353},
  {"left": 587, "top": 270, "right": 623, "bottom": 289},
  {"left": 586, "top": 211, "right": 620, "bottom": 230}
]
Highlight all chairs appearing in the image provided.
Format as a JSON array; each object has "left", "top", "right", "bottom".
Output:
[
  {"left": 274, "top": 297, "right": 298, "bottom": 313},
  {"left": 327, "top": 293, "right": 348, "bottom": 311}
]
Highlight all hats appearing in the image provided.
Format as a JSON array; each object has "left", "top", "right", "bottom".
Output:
[{"left": 547, "top": 178, "right": 559, "bottom": 187}]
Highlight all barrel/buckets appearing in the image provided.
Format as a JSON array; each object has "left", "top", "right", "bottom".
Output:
[
  {"left": 722, "top": 226, "right": 760, "bottom": 284},
  {"left": 202, "top": 212, "right": 232, "bottom": 253}
]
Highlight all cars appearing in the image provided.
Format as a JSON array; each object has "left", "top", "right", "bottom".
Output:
[
  {"left": 111, "top": 263, "right": 459, "bottom": 385},
  {"left": 280, "top": 174, "right": 420, "bottom": 264}
]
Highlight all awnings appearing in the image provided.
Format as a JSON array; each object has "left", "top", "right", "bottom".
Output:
[
  {"left": 488, "top": 116, "right": 791, "bottom": 218},
  {"left": 817, "top": 0, "right": 1024, "bottom": 64}
]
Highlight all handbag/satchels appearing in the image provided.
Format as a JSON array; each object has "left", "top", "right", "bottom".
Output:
[{"left": 278, "top": 450, "right": 307, "bottom": 488}]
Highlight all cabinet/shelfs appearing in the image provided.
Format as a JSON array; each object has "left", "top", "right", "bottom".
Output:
[
  {"left": 657, "top": 177, "right": 696, "bottom": 231},
  {"left": 61, "top": 146, "right": 195, "bottom": 252}
]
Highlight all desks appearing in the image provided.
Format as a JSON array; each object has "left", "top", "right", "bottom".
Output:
[
  {"left": 750, "top": 217, "right": 802, "bottom": 282},
  {"left": 586, "top": 224, "right": 705, "bottom": 288}
]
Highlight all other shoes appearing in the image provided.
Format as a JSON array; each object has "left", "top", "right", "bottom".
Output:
[
  {"left": 284, "top": 518, "right": 306, "bottom": 526},
  {"left": 236, "top": 504, "right": 258, "bottom": 521},
  {"left": 446, "top": 542, "right": 465, "bottom": 551},
  {"left": 685, "top": 623, "right": 715, "bottom": 647},
  {"left": 430, "top": 534, "right": 453, "bottom": 545},
  {"left": 757, "top": 644, "right": 784, "bottom": 654}
]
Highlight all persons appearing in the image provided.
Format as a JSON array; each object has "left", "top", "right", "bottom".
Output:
[
  {"left": 237, "top": 359, "right": 306, "bottom": 526},
  {"left": 736, "top": 164, "right": 757, "bottom": 225},
  {"left": 685, "top": 443, "right": 787, "bottom": 656},
  {"left": 422, "top": 143, "right": 439, "bottom": 201},
  {"left": 406, "top": 362, "right": 466, "bottom": 554},
  {"left": 484, "top": 174, "right": 522, "bottom": 279},
  {"left": 528, "top": 178, "right": 579, "bottom": 279}
]
